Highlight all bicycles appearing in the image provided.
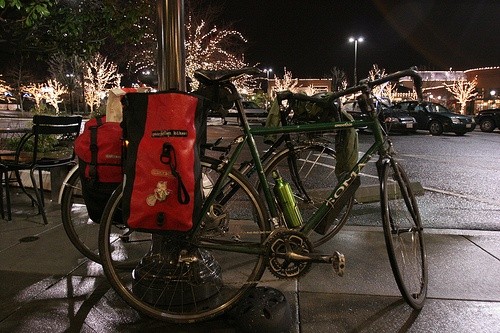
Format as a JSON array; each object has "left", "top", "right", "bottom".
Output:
[
  {"left": 58, "top": 74, "right": 354, "bottom": 270},
  {"left": 98, "top": 67, "right": 428, "bottom": 323}
]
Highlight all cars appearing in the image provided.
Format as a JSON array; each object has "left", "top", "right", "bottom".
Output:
[
  {"left": 390, "top": 101, "right": 476, "bottom": 136},
  {"left": 343, "top": 100, "right": 416, "bottom": 136},
  {"left": 475, "top": 108, "right": 500, "bottom": 133},
  {"left": 222, "top": 101, "right": 267, "bottom": 126}
]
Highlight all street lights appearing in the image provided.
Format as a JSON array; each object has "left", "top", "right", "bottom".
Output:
[
  {"left": 349, "top": 36, "right": 363, "bottom": 85},
  {"left": 263, "top": 69, "right": 273, "bottom": 100}
]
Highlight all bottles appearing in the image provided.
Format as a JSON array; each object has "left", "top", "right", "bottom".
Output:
[{"left": 272, "top": 169, "right": 303, "bottom": 228}]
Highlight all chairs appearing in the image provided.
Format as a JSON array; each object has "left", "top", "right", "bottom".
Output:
[{"left": 0, "top": 115, "right": 82, "bottom": 225}]
